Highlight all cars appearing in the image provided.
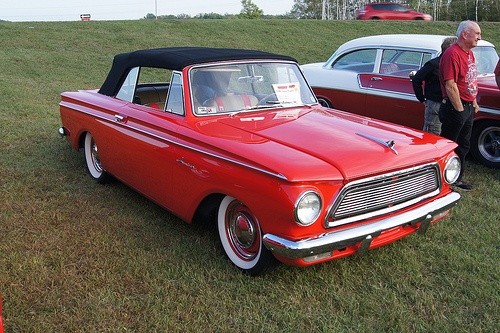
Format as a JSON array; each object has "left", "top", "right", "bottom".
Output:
[
  {"left": 270, "top": 34, "right": 500, "bottom": 167},
  {"left": 58, "top": 46, "right": 462, "bottom": 276}
]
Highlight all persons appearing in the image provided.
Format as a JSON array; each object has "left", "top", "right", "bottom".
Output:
[
  {"left": 412, "top": 36, "right": 457, "bottom": 135},
  {"left": 438, "top": 20, "right": 481, "bottom": 190}
]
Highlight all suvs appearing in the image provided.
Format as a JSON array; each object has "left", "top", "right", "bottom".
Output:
[{"left": 356, "top": 2, "right": 433, "bottom": 21}]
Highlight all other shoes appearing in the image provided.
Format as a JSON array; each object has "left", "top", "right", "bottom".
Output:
[{"left": 453, "top": 183, "right": 476, "bottom": 191}]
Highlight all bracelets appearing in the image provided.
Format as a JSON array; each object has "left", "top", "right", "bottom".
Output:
[{"left": 473, "top": 103, "right": 477, "bottom": 107}]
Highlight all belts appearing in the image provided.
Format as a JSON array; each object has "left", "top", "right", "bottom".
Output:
[{"left": 462, "top": 102, "right": 473, "bottom": 106}]
